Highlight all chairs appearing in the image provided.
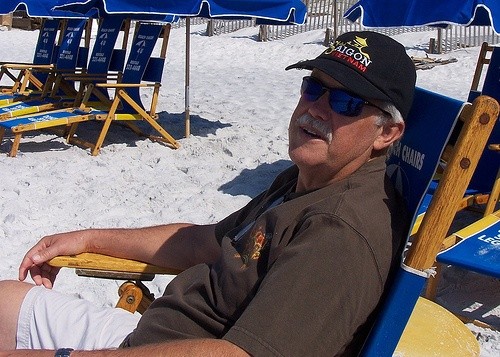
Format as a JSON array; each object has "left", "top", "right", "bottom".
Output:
[{"left": 0, "top": 18, "right": 500, "bottom": 357}]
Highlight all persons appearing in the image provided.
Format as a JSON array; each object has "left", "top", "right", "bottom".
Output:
[{"left": 0, "top": 30, "right": 417, "bottom": 357}]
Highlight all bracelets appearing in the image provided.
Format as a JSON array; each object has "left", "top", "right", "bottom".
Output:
[{"left": 53, "top": 348, "right": 74, "bottom": 357}]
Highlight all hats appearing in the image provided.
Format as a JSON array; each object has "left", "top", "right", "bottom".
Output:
[{"left": 285, "top": 31, "right": 416, "bottom": 122}]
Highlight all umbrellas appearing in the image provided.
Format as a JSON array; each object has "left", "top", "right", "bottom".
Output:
[
  {"left": 343, "top": 0, "right": 500, "bottom": 37},
  {"left": 0, "top": 0, "right": 308, "bottom": 138}
]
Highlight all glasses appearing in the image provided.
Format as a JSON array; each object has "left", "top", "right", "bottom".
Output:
[
  {"left": 300, "top": 75, "right": 392, "bottom": 117},
  {"left": 233, "top": 195, "right": 285, "bottom": 242}
]
